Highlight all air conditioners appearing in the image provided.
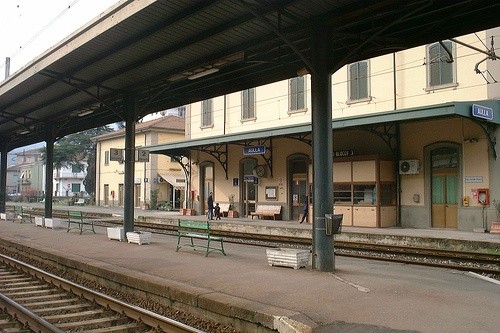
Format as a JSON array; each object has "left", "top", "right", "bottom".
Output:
[
  {"left": 158, "top": 176, "right": 165, "bottom": 184},
  {"left": 398, "top": 160, "right": 419, "bottom": 175}
]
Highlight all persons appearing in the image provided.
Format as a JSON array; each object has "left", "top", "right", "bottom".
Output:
[{"left": 207, "top": 192, "right": 221, "bottom": 219}]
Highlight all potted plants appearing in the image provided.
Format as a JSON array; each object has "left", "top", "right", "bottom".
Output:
[
  {"left": 186, "top": 198, "right": 196, "bottom": 216},
  {"left": 227, "top": 194, "right": 238, "bottom": 218},
  {"left": 490, "top": 198, "right": 500, "bottom": 233}
]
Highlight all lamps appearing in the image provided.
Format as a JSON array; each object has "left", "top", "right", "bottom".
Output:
[
  {"left": 187, "top": 67, "right": 219, "bottom": 80},
  {"left": 77, "top": 110, "right": 94, "bottom": 116}
]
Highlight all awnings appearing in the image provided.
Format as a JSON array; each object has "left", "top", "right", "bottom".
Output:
[{"left": 147, "top": 98, "right": 500, "bottom": 161}]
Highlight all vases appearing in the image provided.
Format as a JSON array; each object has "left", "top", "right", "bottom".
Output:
[
  {"left": 107, "top": 226, "right": 124, "bottom": 242},
  {"left": 45, "top": 218, "right": 67, "bottom": 229},
  {"left": 126, "top": 230, "right": 152, "bottom": 246},
  {"left": 0, "top": 211, "right": 15, "bottom": 221},
  {"left": 35, "top": 216, "right": 45, "bottom": 228},
  {"left": 266, "top": 248, "right": 310, "bottom": 269}
]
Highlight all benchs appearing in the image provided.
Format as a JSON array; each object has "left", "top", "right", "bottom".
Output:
[
  {"left": 13, "top": 205, "right": 32, "bottom": 224},
  {"left": 67, "top": 210, "right": 97, "bottom": 235},
  {"left": 250, "top": 204, "right": 282, "bottom": 221},
  {"left": 204, "top": 203, "right": 231, "bottom": 217},
  {"left": 175, "top": 218, "right": 226, "bottom": 257},
  {"left": 74, "top": 199, "right": 84, "bottom": 206}
]
